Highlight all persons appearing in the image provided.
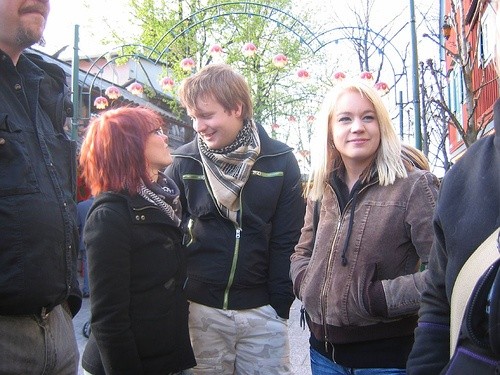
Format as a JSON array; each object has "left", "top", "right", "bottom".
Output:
[
  {"left": 78, "top": 106, "right": 198, "bottom": 375},
  {"left": 289, "top": 76, "right": 441, "bottom": 375},
  {"left": 404, "top": 97, "right": 500, "bottom": 375},
  {"left": 162, "top": 63, "right": 306, "bottom": 375},
  {"left": 0, "top": 0, "right": 84, "bottom": 375}
]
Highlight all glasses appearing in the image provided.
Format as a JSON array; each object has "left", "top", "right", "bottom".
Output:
[{"left": 146, "top": 129, "right": 163, "bottom": 135}]
